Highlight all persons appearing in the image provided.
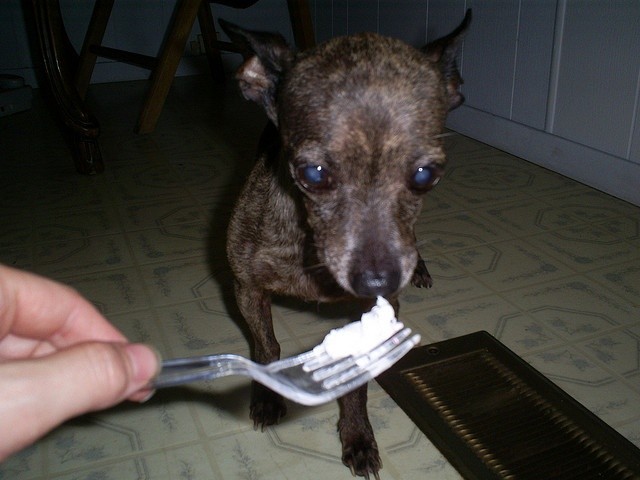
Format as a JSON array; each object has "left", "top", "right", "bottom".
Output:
[{"left": 0, "top": 265, "right": 163, "bottom": 468}]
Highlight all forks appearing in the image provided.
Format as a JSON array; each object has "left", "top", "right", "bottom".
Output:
[{"left": 141, "top": 307, "right": 420, "bottom": 407}]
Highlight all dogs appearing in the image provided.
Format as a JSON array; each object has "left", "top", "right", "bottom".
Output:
[{"left": 215, "top": 7, "right": 473, "bottom": 480}]
